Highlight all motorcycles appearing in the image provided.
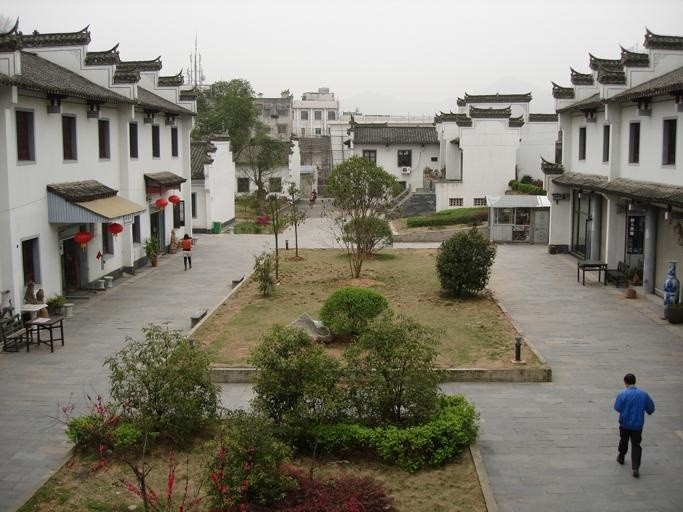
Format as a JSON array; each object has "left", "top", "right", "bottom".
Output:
[{"left": 309, "top": 193, "right": 315, "bottom": 202}]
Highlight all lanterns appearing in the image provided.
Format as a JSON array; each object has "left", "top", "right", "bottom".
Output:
[
  {"left": 154, "top": 198, "right": 167, "bottom": 215},
  {"left": 73, "top": 230, "right": 93, "bottom": 252},
  {"left": 168, "top": 195, "right": 180, "bottom": 209},
  {"left": 105, "top": 222, "right": 123, "bottom": 242}
]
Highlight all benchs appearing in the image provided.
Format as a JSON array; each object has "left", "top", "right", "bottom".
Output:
[
  {"left": 0, "top": 313, "right": 32, "bottom": 353},
  {"left": 188, "top": 306, "right": 210, "bottom": 329},
  {"left": 602, "top": 260, "right": 631, "bottom": 288},
  {"left": 230, "top": 271, "right": 246, "bottom": 290}
]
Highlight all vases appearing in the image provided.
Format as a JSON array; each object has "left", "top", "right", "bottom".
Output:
[
  {"left": 661, "top": 257, "right": 682, "bottom": 320},
  {"left": 665, "top": 303, "right": 682, "bottom": 325}
]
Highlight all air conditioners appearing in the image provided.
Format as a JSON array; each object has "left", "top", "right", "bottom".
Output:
[{"left": 400, "top": 165, "right": 412, "bottom": 176}]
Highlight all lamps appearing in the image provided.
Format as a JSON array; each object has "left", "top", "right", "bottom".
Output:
[
  {"left": 577, "top": 188, "right": 583, "bottom": 198},
  {"left": 385, "top": 143, "right": 388, "bottom": 150},
  {"left": 420, "top": 143, "right": 424, "bottom": 151},
  {"left": 627, "top": 199, "right": 639, "bottom": 212},
  {"left": 662, "top": 202, "right": 673, "bottom": 220}
]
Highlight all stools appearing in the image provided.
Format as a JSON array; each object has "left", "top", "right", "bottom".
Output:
[
  {"left": 98, "top": 278, "right": 107, "bottom": 290},
  {"left": 62, "top": 301, "right": 75, "bottom": 320},
  {"left": 104, "top": 276, "right": 115, "bottom": 289}
]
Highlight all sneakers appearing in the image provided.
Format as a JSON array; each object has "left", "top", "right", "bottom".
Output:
[
  {"left": 616, "top": 454, "right": 626, "bottom": 466},
  {"left": 632, "top": 470, "right": 641, "bottom": 480}
]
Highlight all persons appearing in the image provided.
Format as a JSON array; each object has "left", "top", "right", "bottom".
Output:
[
  {"left": 326, "top": 199, "right": 331, "bottom": 214},
  {"left": 308, "top": 189, "right": 316, "bottom": 208},
  {"left": 613, "top": 373, "right": 655, "bottom": 477},
  {"left": 320, "top": 199, "right": 325, "bottom": 218},
  {"left": 181, "top": 233, "right": 192, "bottom": 271}
]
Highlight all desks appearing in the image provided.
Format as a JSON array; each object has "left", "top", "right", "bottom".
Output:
[
  {"left": 20, "top": 301, "right": 46, "bottom": 323},
  {"left": 24, "top": 316, "right": 65, "bottom": 354},
  {"left": 576, "top": 260, "right": 608, "bottom": 286}
]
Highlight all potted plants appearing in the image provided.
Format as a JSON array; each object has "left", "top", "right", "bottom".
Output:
[
  {"left": 141, "top": 233, "right": 161, "bottom": 266},
  {"left": 47, "top": 291, "right": 66, "bottom": 316}
]
